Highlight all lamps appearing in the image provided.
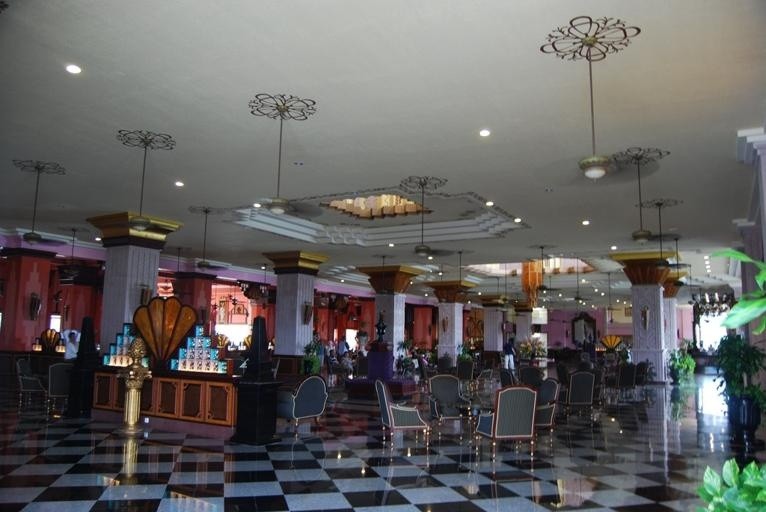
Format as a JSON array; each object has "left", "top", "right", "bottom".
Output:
[
  {"left": 23, "top": 160, "right": 44, "bottom": 247},
  {"left": 414, "top": 180, "right": 430, "bottom": 257},
  {"left": 127, "top": 139, "right": 153, "bottom": 232},
  {"left": 198, "top": 208, "right": 211, "bottom": 271},
  {"left": 574, "top": 35, "right": 688, "bottom": 292},
  {"left": 268, "top": 105, "right": 293, "bottom": 216}
]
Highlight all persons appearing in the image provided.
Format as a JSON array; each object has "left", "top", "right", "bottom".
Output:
[
  {"left": 64, "top": 331, "right": 79, "bottom": 362},
  {"left": 323, "top": 325, "right": 438, "bottom": 388},
  {"left": 267, "top": 336, "right": 275, "bottom": 350},
  {"left": 503, "top": 338, "right": 517, "bottom": 371}
]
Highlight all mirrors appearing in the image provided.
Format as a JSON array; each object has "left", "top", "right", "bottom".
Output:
[{"left": 572, "top": 312, "right": 597, "bottom": 347}]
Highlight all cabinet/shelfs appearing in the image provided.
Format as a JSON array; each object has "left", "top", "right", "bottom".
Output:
[{"left": 90, "top": 323, "right": 238, "bottom": 443}]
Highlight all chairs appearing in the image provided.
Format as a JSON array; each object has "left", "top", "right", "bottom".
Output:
[
  {"left": 276, "top": 375, "right": 328, "bottom": 440},
  {"left": 374, "top": 357, "right": 648, "bottom": 465},
  {"left": 326, "top": 356, "right": 368, "bottom": 385},
  {"left": 15, "top": 357, "right": 74, "bottom": 421}
]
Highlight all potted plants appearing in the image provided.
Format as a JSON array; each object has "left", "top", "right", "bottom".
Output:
[
  {"left": 729, "top": 382, "right": 765, "bottom": 448},
  {"left": 668, "top": 351, "right": 697, "bottom": 385}
]
